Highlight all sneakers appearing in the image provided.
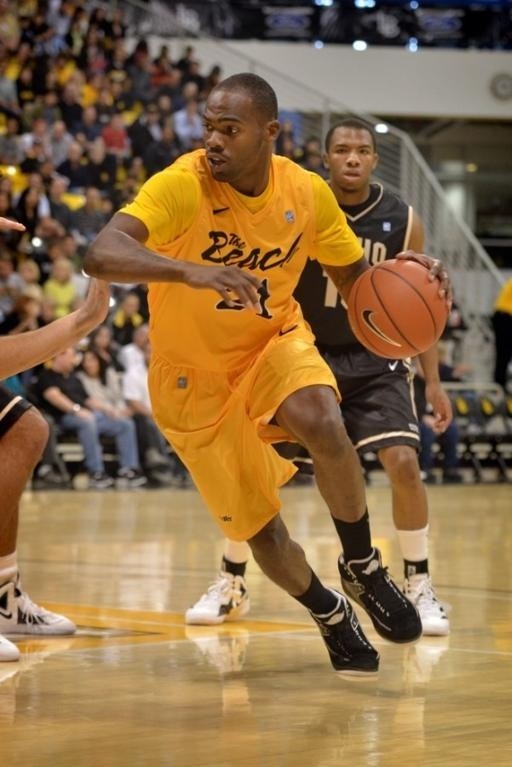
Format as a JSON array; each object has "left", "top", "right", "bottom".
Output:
[
  {"left": 306, "top": 584, "right": 379, "bottom": 676},
  {"left": 183, "top": 568, "right": 250, "bottom": 625},
  {"left": 336, "top": 546, "right": 422, "bottom": 643},
  {"left": 85, "top": 467, "right": 148, "bottom": 490},
  {"left": 0, "top": 572, "right": 77, "bottom": 664},
  {"left": 401, "top": 569, "right": 450, "bottom": 638}
]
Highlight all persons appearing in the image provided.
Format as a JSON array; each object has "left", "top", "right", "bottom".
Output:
[
  {"left": 1, "top": 1, "right": 511, "bottom": 486},
  {"left": 85, "top": 73, "right": 452, "bottom": 675},
  {"left": 183, "top": 119, "right": 449, "bottom": 636},
  {"left": 2, "top": 220, "right": 112, "bottom": 660}
]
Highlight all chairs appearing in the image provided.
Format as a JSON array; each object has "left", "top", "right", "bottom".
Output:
[{"left": 30, "top": 375, "right": 511, "bottom": 485}]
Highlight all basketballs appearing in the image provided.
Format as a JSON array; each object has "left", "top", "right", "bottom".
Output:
[{"left": 348, "top": 259, "right": 450, "bottom": 360}]
